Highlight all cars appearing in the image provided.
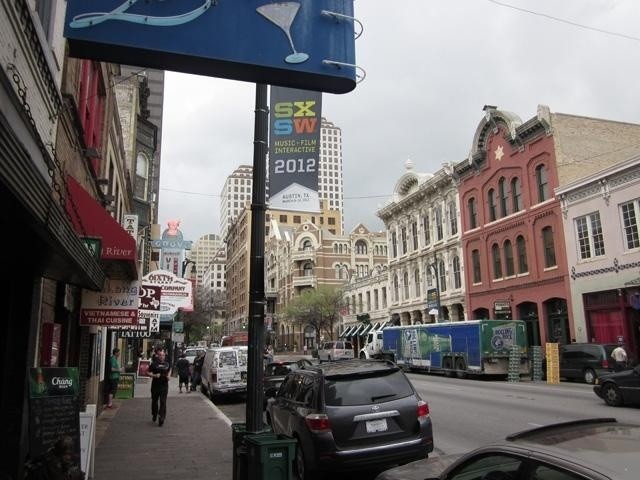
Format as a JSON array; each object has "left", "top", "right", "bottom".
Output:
[
  {"left": 424, "top": 418, "right": 640, "bottom": 480},
  {"left": 593, "top": 364, "right": 640, "bottom": 407}
]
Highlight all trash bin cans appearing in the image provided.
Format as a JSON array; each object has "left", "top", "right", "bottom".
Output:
[{"left": 231, "top": 422, "right": 297, "bottom": 480}]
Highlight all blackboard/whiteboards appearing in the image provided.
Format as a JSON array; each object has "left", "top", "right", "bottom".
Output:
[{"left": 27, "top": 366, "right": 94, "bottom": 480}]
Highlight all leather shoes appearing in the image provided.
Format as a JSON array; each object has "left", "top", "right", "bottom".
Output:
[
  {"left": 160, "top": 416, "right": 164, "bottom": 424},
  {"left": 153, "top": 415, "right": 156, "bottom": 421}
]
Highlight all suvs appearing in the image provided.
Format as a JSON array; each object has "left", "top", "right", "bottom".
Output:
[{"left": 263, "top": 358, "right": 433, "bottom": 479}]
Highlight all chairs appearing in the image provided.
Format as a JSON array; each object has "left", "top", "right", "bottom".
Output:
[{"left": 275, "top": 367, "right": 289, "bottom": 375}]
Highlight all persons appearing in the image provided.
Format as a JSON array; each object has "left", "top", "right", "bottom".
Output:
[
  {"left": 145, "top": 348, "right": 170, "bottom": 426},
  {"left": 190, "top": 350, "right": 206, "bottom": 391},
  {"left": 610, "top": 342, "right": 627, "bottom": 372},
  {"left": 267, "top": 344, "right": 274, "bottom": 363},
  {"left": 176, "top": 354, "right": 191, "bottom": 393},
  {"left": 304, "top": 345, "right": 308, "bottom": 355},
  {"left": 106, "top": 348, "right": 124, "bottom": 408}
]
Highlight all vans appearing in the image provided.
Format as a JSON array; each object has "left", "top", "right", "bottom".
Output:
[
  {"left": 541, "top": 342, "right": 627, "bottom": 383},
  {"left": 179, "top": 332, "right": 248, "bottom": 402},
  {"left": 317, "top": 339, "right": 355, "bottom": 363}
]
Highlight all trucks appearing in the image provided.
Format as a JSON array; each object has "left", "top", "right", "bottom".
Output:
[{"left": 360, "top": 319, "right": 530, "bottom": 380}]
